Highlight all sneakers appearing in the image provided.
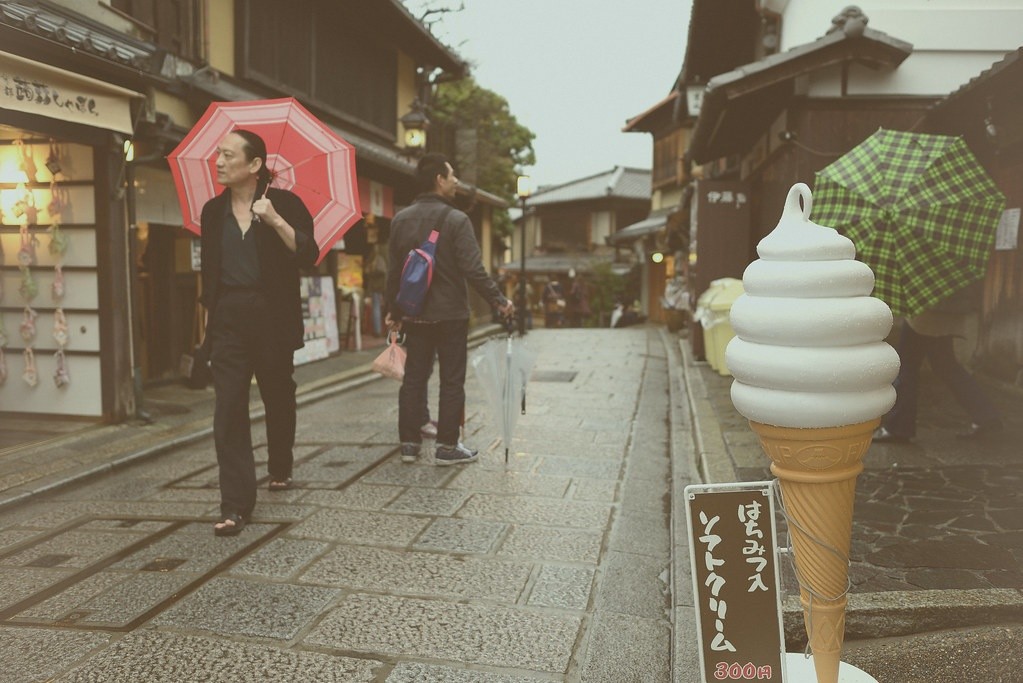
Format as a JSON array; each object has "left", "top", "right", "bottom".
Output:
[
  {"left": 436, "top": 444, "right": 480, "bottom": 467},
  {"left": 400, "top": 444, "right": 420, "bottom": 463}
]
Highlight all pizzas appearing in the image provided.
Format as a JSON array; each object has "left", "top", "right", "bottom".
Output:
[{"left": 726, "top": 183, "right": 900, "bottom": 683}]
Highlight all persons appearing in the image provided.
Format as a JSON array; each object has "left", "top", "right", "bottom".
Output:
[
  {"left": 364, "top": 243, "right": 388, "bottom": 339},
  {"left": 387, "top": 152, "right": 516, "bottom": 466},
  {"left": 195, "top": 129, "right": 320, "bottom": 537},
  {"left": 869, "top": 312, "right": 1005, "bottom": 443},
  {"left": 513, "top": 278, "right": 535, "bottom": 330},
  {"left": 568, "top": 274, "right": 590, "bottom": 328},
  {"left": 542, "top": 274, "right": 564, "bottom": 328},
  {"left": 613, "top": 291, "right": 632, "bottom": 313}
]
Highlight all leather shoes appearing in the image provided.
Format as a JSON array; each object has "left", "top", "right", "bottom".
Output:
[
  {"left": 872, "top": 426, "right": 912, "bottom": 443},
  {"left": 958, "top": 420, "right": 1007, "bottom": 440}
]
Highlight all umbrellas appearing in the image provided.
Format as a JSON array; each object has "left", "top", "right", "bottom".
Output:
[
  {"left": 809, "top": 125, "right": 1008, "bottom": 318},
  {"left": 164, "top": 93, "right": 364, "bottom": 267}
]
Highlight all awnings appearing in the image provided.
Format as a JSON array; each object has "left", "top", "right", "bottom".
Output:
[{"left": 611, "top": 216, "right": 668, "bottom": 239}]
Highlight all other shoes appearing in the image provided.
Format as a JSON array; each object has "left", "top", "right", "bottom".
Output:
[{"left": 419, "top": 418, "right": 438, "bottom": 438}]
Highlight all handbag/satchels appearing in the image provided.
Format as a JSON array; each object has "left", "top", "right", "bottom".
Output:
[
  {"left": 373, "top": 327, "right": 407, "bottom": 381},
  {"left": 397, "top": 204, "right": 453, "bottom": 319}
]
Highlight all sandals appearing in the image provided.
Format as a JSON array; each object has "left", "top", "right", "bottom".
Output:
[
  {"left": 216, "top": 513, "right": 247, "bottom": 535},
  {"left": 269, "top": 475, "right": 293, "bottom": 490}
]
